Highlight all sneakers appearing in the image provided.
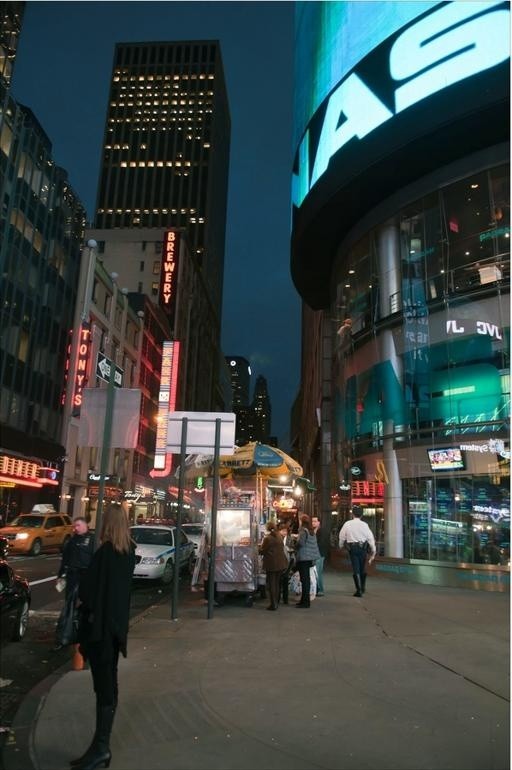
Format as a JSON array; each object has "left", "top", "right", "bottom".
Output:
[
  {"left": 50, "top": 644, "right": 64, "bottom": 651},
  {"left": 266, "top": 598, "right": 313, "bottom": 611}
]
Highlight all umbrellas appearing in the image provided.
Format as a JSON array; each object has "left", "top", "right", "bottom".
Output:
[
  {"left": 210, "top": 440, "right": 305, "bottom": 523},
  {"left": 174, "top": 444, "right": 251, "bottom": 500}
]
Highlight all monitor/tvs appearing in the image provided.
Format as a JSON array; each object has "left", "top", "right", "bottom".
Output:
[
  {"left": 426, "top": 446, "right": 467, "bottom": 471},
  {"left": 495, "top": 441, "right": 510, "bottom": 469}
]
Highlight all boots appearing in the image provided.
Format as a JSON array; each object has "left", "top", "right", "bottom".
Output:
[
  {"left": 68, "top": 689, "right": 118, "bottom": 770},
  {"left": 351, "top": 571, "right": 369, "bottom": 598}
]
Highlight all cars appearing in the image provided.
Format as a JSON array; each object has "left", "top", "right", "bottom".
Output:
[
  {"left": 118, "top": 524, "right": 208, "bottom": 586},
  {"left": 0, "top": 504, "right": 76, "bottom": 557},
  {"left": 0, "top": 560, "right": 32, "bottom": 642}
]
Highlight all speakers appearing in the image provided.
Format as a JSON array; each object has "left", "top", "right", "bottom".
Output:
[{"left": 352, "top": 460, "right": 365, "bottom": 481}]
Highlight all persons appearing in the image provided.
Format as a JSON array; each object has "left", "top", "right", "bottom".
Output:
[
  {"left": 337, "top": 506, "right": 377, "bottom": 598},
  {"left": 258, "top": 506, "right": 331, "bottom": 610},
  {"left": 51, "top": 517, "right": 95, "bottom": 650},
  {"left": 137, "top": 513, "right": 192, "bottom": 525},
  {"left": 69, "top": 503, "right": 137, "bottom": 770},
  {"left": 8, "top": 500, "right": 23, "bottom": 527}
]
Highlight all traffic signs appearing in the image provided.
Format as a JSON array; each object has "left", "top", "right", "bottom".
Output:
[{"left": 95, "top": 350, "right": 125, "bottom": 389}]
